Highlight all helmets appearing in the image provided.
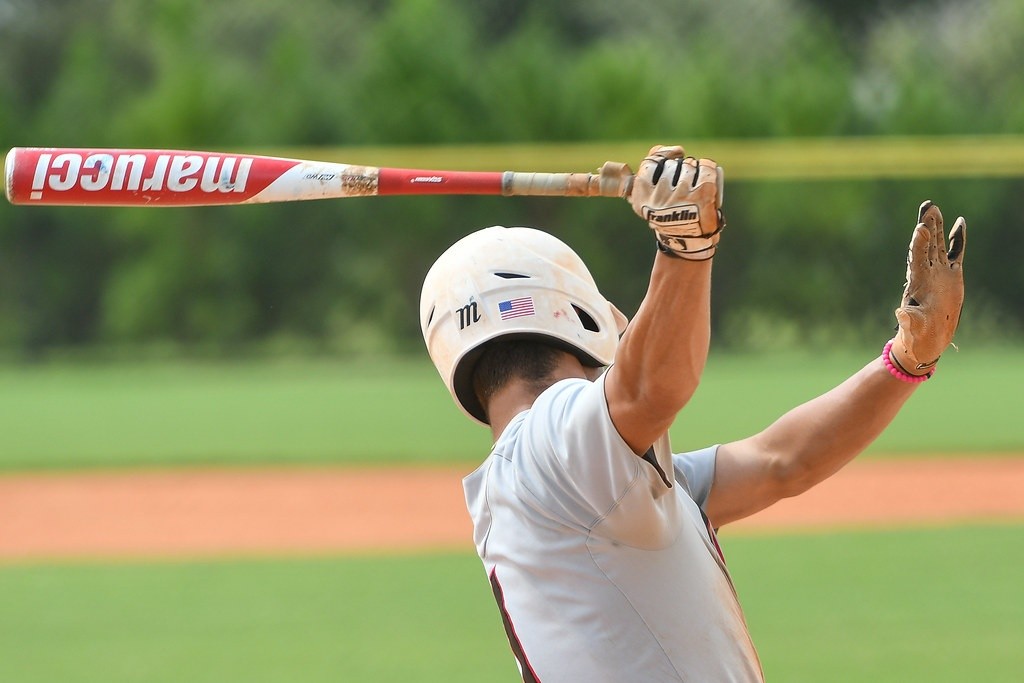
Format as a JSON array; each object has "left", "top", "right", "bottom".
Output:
[{"left": 418, "top": 225, "right": 629, "bottom": 429}]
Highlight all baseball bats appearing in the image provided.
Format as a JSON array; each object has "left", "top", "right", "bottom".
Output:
[{"left": 4, "top": 145, "right": 725, "bottom": 209}]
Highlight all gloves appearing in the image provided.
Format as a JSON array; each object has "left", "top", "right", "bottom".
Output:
[
  {"left": 626, "top": 144, "right": 727, "bottom": 261},
  {"left": 888, "top": 199, "right": 967, "bottom": 377}
]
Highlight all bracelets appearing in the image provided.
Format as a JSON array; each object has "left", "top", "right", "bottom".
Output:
[{"left": 881, "top": 339, "right": 943, "bottom": 382}]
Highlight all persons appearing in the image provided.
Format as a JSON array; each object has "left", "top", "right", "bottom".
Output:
[{"left": 420, "top": 144, "right": 966, "bottom": 683}]
